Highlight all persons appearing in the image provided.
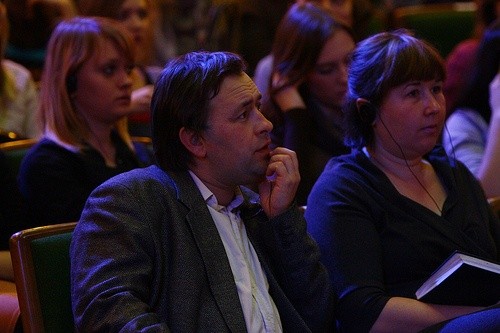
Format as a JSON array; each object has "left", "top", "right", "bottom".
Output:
[
  {"left": 254, "top": 0, "right": 353, "bottom": 101},
  {"left": 69, "top": 52, "right": 337, "bottom": 333},
  {"left": 264, "top": 0, "right": 356, "bottom": 204},
  {"left": 305, "top": 32, "right": 500, "bottom": 333},
  {"left": 12, "top": 16, "right": 137, "bottom": 227},
  {"left": 0, "top": 0, "right": 171, "bottom": 141},
  {"left": 443, "top": 0, "right": 500, "bottom": 110},
  {"left": 442, "top": 21, "right": 500, "bottom": 199}
]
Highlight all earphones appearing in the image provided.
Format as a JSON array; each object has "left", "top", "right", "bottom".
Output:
[{"left": 358, "top": 102, "right": 376, "bottom": 125}]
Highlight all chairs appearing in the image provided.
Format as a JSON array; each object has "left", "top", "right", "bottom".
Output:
[
  {"left": 0, "top": 135, "right": 41, "bottom": 250},
  {"left": 8, "top": 220, "right": 78, "bottom": 333}
]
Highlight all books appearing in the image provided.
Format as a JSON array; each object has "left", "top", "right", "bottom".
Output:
[{"left": 414, "top": 250, "right": 500, "bottom": 305}]
